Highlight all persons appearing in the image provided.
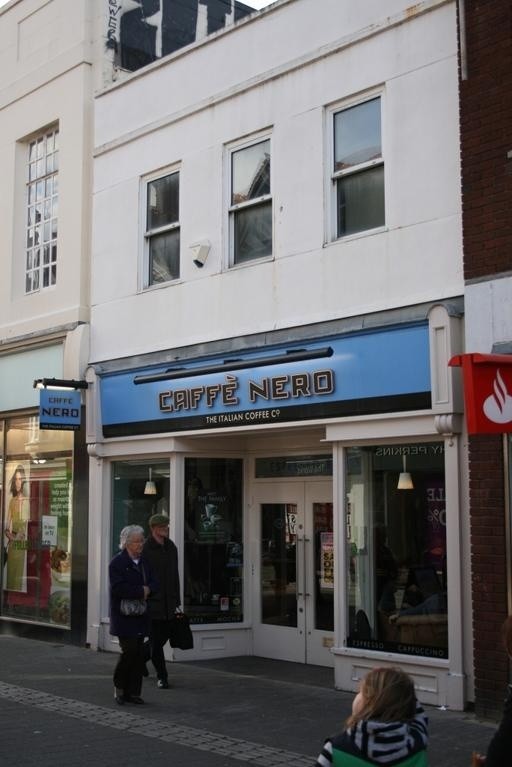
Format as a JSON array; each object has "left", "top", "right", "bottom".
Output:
[
  {"left": 482, "top": 677, "right": 512, "bottom": 767},
  {"left": 106, "top": 524, "right": 152, "bottom": 708},
  {"left": 314, "top": 663, "right": 430, "bottom": 767},
  {"left": 138, "top": 514, "right": 182, "bottom": 689},
  {"left": 371, "top": 520, "right": 447, "bottom": 625},
  {"left": 3, "top": 463, "right": 29, "bottom": 592}
]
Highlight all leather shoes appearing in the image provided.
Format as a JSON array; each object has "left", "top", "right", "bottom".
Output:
[
  {"left": 114, "top": 686, "right": 144, "bottom": 705},
  {"left": 143, "top": 662, "right": 149, "bottom": 678},
  {"left": 157, "top": 678, "right": 168, "bottom": 688}
]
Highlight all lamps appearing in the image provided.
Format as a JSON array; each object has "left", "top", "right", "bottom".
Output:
[
  {"left": 396, "top": 455, "right": 415, "bottom": 490},
  {"left": 143, "top": 467, "right": 157, "bottom": 495}
]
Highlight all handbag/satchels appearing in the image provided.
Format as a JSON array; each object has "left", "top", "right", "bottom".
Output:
[
  {"left": 122, "top": 598, "right": 149, "bottom": 616},
  {"left": 169, "top": 613, "right": 193, "bottom": 650}
]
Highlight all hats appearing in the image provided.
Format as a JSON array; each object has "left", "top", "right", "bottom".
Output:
[{"left": 149, "top": 514, "right": 170, "bottom": 527}]
